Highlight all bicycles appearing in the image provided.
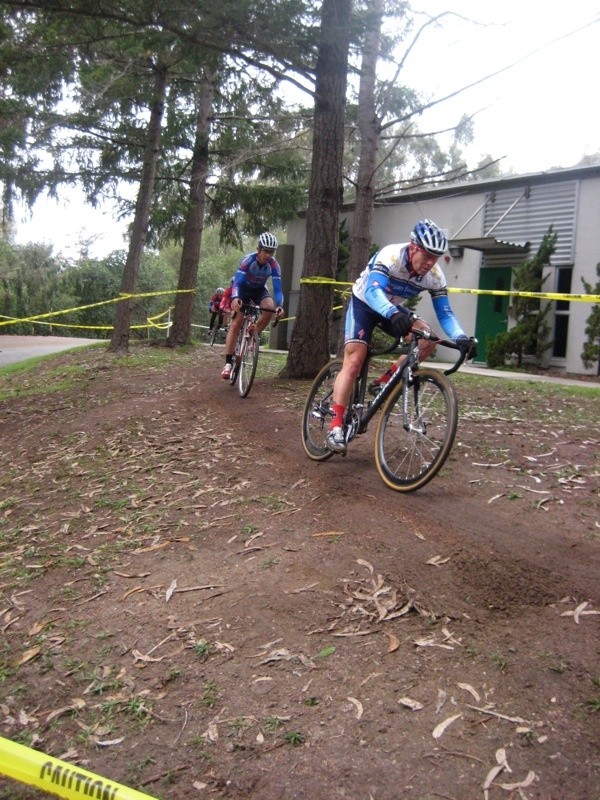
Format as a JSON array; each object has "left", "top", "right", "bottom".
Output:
[
  {"left": 300, "top": 305, "right": 481, "bottom": 493},
  {"left": 207, "top": 310, "right": 228, "bottom": 347},
  {"left": 221, "top": 299, "right": 282, "bottom": 398}
]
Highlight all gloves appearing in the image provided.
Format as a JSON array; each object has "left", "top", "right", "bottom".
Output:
[
  {"left": 391, "top": 311, "right": 412, "bottom": 337},
  {"left": 458, "top": 336, "right": 477, "bottom": 359}
]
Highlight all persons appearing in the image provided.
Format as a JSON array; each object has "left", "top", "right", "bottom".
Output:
[
  {"left": 221, "top": 232, "right": 284, "bottom": 379},
  {"left": 207, "top": 276, "right": 235, "bottom": 335},
  {"left": 323, "top": 219, "right": 478, "bottom": 454}
]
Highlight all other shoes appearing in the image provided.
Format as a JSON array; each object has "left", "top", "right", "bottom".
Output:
[
  {"left": 208, "top": 330, "right": 211, "bottom": 335},
  {"left": 248, "top": 335, "right": 255, "bottom": 351},
  {"left": 221, "top": 363, "right": 232, "bottom": 378},
  {"left": 217, "top": 330, "right": 220, "bottom": 334}
]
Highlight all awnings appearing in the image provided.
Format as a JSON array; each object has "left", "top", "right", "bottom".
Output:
[{"left": 448, "top": 237, "right": 531, "bottom": 252}]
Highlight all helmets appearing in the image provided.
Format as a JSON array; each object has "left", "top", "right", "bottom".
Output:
[
  {"left": 410, "top": 218, "right": 448, "bottom": 256},
  {"left": 230, "top": 276, "right": 235, "bottom": 283},
  {"left": 260, "top": 232, "right": 278, "bottom": 251},
  {"left": 216, "top": 288, "right": 225, "bottom": 295}
]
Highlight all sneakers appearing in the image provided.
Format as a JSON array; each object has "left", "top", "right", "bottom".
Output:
[
  {"left": 324, "top": 426, "right": 347, "bottom": 454},
  {"left": 368, "top": 382, "right": 383, "bottom": 398}
]
